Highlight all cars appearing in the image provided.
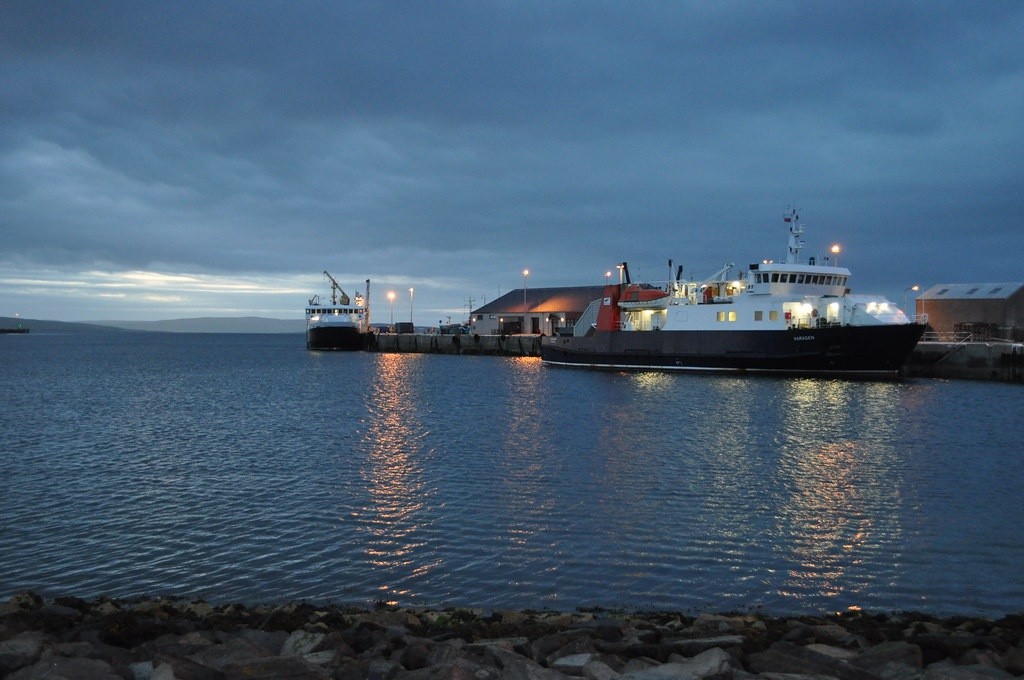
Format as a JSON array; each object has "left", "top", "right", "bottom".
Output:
[{"left": 449, "top": 327, "right": 469, "bottom": 334}]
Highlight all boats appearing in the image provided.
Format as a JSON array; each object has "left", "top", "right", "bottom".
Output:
[
  {"left": 538, "top": 199, "right": 930, "bottom": 376},
  {"left": 305, "top": 271, "right": 365, "bottom": 352}
]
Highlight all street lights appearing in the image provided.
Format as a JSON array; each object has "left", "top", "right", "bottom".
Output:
[
  {"left": 904, "top": 285, "right": 918, "bottom": 314},
  {"left": 523, "top": 270, "right": 529, "bottom": 313},
  {"left": 410, "top": 288, "right": 414, "bottom": 322},
  {"left": 387, "top": 291, "right": 395, "bottom": 324},
  {"left": 605, "top": 271, "right": 611, "bottom": 284}
]
